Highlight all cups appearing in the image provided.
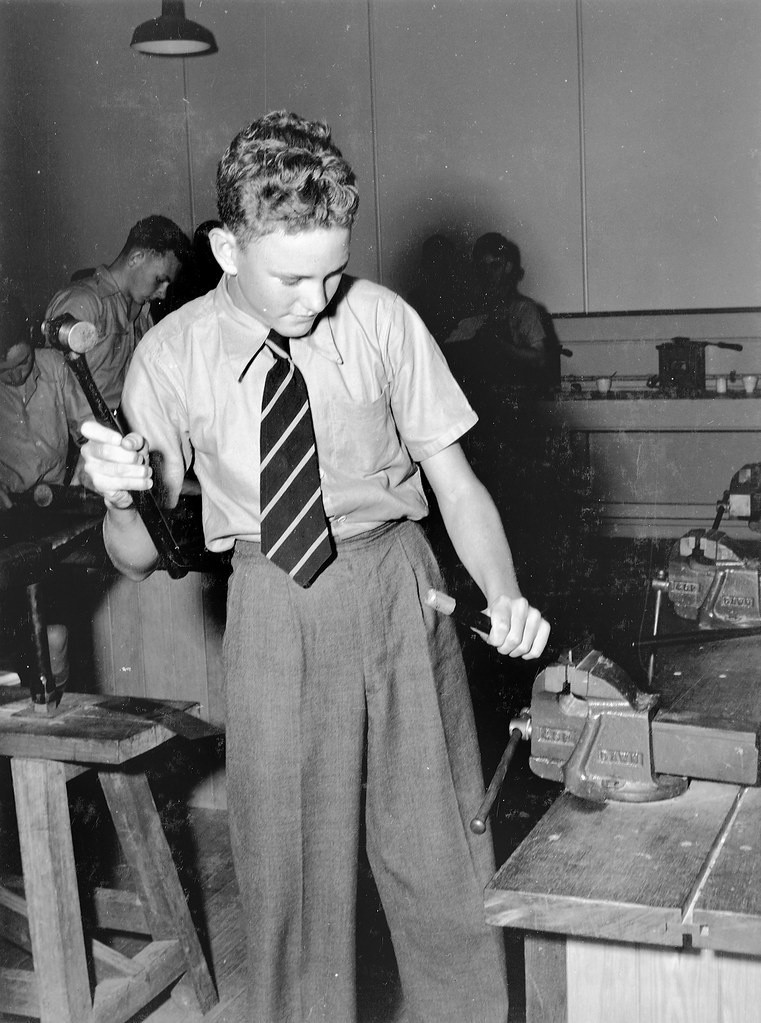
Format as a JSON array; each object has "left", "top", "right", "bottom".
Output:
[{"left": 716, "top": 378, "right": 727, "bottom": 394}]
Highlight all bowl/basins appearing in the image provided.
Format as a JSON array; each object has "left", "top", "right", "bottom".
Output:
[
  {"left": 596, "top": 378, "right": 611, "bottom": 394},
  {"left": 742, "top": 375, "right": 757, "bottom": 393}
]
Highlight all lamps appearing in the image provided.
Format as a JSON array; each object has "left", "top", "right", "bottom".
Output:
[{"left": 129, "top": 1, "right": 216, "bottom": 59}]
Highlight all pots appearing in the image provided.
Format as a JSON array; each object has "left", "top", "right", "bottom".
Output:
[{"left": 654, "top": 337, "right": 742, "bottom": 396}]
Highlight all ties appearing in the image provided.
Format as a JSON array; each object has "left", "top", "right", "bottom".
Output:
[{"left": 258, "top": 329, "right": 334, "bottom": 588}]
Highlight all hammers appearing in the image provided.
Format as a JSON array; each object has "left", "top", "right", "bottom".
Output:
[{"left": 28, "top": 311, "right": 188, "bottom": 582}]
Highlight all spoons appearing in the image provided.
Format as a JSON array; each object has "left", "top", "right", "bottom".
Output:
[{"left": 609, "top": 371, "right": 617, "bottom": 379}]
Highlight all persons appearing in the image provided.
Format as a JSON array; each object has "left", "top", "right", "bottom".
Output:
[
  {"left": 0, "top": 300, "right": 100, "bottom": 696},
  {"left": 468, "top": 231, "right": 563, "bottom": 448},
  {"left": 405, "top": 234, "right": 480, "bottom": 337},
  {"left": 78, "top": 112, "right": 550, "bottom": 1023},
  {"left": 45, "top": 216, "right": 195, "bottom": 449}
]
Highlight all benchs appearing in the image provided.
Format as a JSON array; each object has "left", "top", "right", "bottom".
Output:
[{"left": 0, "top": 683, "right": 221, "bottom": 1023}]
